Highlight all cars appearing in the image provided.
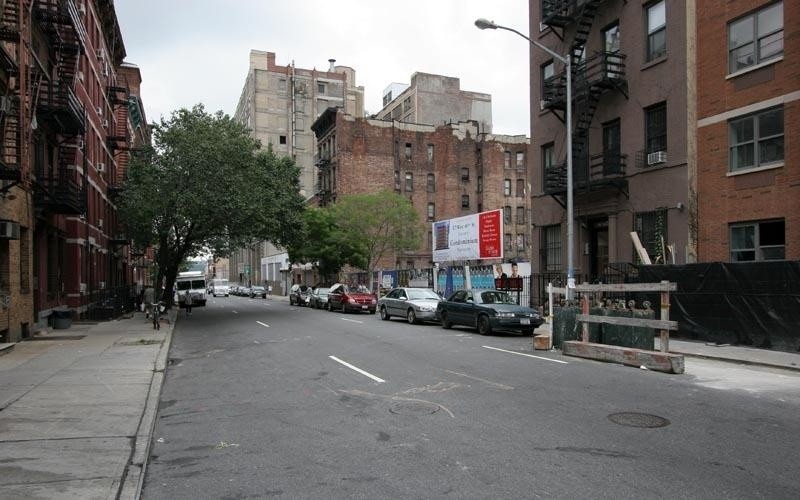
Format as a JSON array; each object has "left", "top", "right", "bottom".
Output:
[
  {"left": 436, "top": 288, "right": 547, "bottom": 337},
  {"left": 207, "top": 281, "right": 213, "bottom": 294},
  {"left": 328, "top": 282, "right": 377, "bottom": 315},
  {"left": 377, "top": 287, "right": 447, "bottom": 325},
  {"left": 288, "top": 283, "right": 315, "bottom": 307},
  {"left": 309, "top": 287, "right": 330, "bottom": 309},
  {"left": 229, "top": 285, "right": 250, "bottom": 297}
]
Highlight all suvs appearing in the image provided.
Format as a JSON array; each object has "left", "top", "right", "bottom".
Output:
[{"left": 249, "top": 285, "right": 267, "bottom": 298}]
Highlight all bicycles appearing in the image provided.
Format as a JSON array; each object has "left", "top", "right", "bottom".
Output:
[{"left": 149, "top": 299, "right": 165, "bottom": 330}]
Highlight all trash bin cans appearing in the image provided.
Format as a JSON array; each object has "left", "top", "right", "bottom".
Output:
[{"left": 545, "top": 298, "right": 655, "bottom": 351}]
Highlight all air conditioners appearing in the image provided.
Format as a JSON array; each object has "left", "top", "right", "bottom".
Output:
[
  {"left": 96, "top": 49, "right": 104, "bottom": 59},
  {"left": 79, "top": 141, "right": 84, "bottom": 148},
  {"left": 0, "top": 222, "right": 21, "bottom": 240},
  {"left": 103, "top": 120, "right": 109, "bottom": 127},
  {"left": 96, "top": 107, "right": 103, "bottom": 115},
  {"left": 78, "top": 3, "right": 86, "bottom": 15},
  {"left": 97, "top": 218, "right": 103, "bottom": 228},
  {"left": 97, "top": 163, "right": 105, "bottom": 172},
  {"left": 80, "top": 283, "right": 87, "bottom": 292},
  {"left": 77, "top": 72, "right": 84, "bottom": 82},
  {"left": 647, "top": 152, "right": 668, "bottom": 166},
  {"left": 98, "top": 281, "right": 106, "bottom": 290}
]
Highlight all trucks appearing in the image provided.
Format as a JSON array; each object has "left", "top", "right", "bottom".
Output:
[
  {"left": 213, "top": 279, "right": 229, "bottom": 297},
  {"left": 175, "top": 270, "right": 207, "bottom": 307}
]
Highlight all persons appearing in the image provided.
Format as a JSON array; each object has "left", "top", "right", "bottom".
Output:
[
  {"left": 143, "top": 287, "right": 156, "bottom": 324},
  {"left": 496, "top": 264, "right": 507, "bottom": 279},
  {"left": 264, "top": 280, "right": 269, "bottom": 294},
  {"left": 184, "top": 291, "right": 194, "bottom": 316},
  {"left": 268, "top": 285, "right": 273, "bottom": 296},
  {"left": 510, "top": 262, "right": 522, "bottom": 278}
]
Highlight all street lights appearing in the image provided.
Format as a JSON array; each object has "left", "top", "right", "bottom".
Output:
[{"left": 474, "top": 19, "right": 579, "bottom": 306}]
